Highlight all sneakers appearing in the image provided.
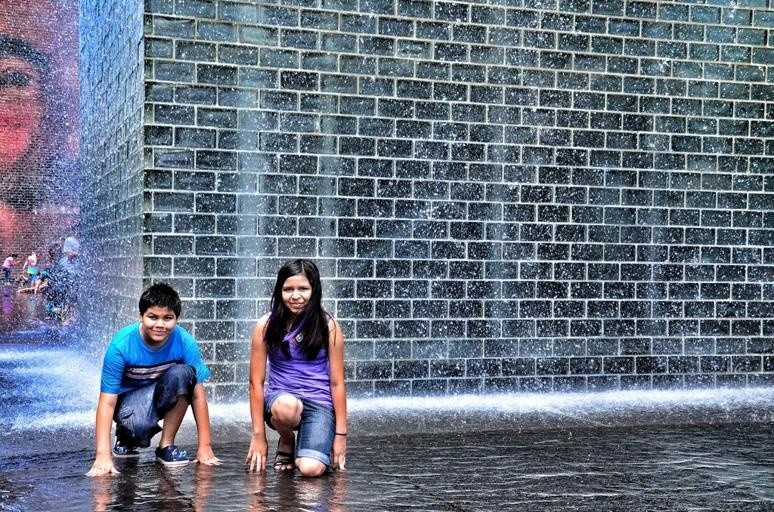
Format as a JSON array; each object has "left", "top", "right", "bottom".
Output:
[
  {"left": 154, "top": 445, "right": 189, "bottom": 468},
  {"left": 111, "top": 436, "right": 139, "bottom": 458}
]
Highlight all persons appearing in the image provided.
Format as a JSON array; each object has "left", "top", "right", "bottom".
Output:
[
  {"left": 241, "top": 471, "right": 350, "bottom": 511},
  {"left": 242, "top": 258, "right": 350, "bottom": 477},
  {"left": 0, "top": 219, "right": 79, "bottom": 329},
  {"left": 90, "top": 457, "right": 214, "bottom": 510},
  {"left": 82, "top": 281, "right": 226, "bottom": 480},
  {"left": 2, "top": 34, "right": 69, "bottom": 220}
]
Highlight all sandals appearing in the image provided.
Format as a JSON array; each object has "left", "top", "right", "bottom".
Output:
[{"left": 273, "top": 433, "right": 295, "bottom": 472}]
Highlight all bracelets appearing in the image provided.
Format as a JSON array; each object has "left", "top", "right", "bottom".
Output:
[{"left": 333, "top": 431, "right": 349, "bottom": 438}]
